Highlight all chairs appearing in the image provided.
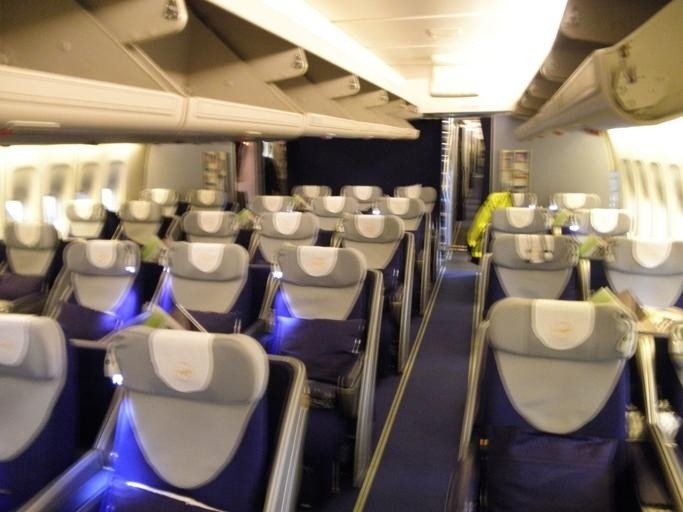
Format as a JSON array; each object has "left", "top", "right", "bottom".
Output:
[
  {"left": 458, "top": 188, "right": 682, "bottom": 512},
  {"left": 2, "top": 188, "right": 438, "bottom": 511}
]
